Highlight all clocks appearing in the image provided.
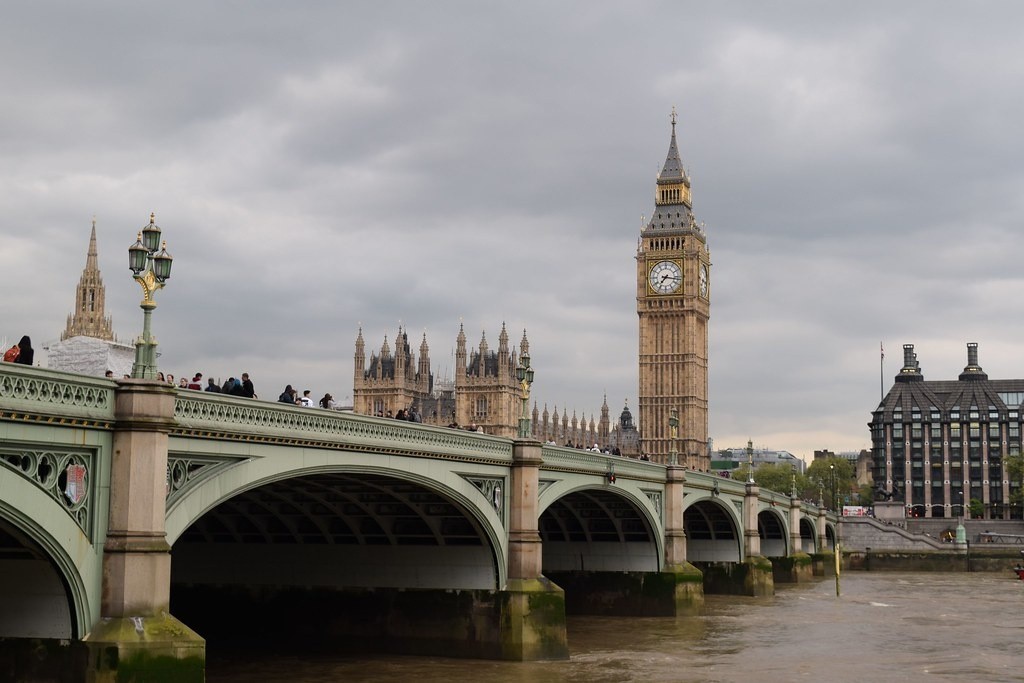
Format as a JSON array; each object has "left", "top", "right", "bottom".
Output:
[
  {"left": 647, "top": 259, "right": 684, "bottom": 295},
  {"left": 698, "top": 263, "right": 708, "bottom": 299}
]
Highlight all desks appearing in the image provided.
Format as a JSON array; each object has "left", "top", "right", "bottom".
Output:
[{"left": 980, "top": 533, "right": 1024, "bottom": 543}]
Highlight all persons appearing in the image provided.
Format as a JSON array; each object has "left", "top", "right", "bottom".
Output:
[
  {"left": 690, "top": 464, "right": 730, "bottom": 479},
  {"left": 3, "top": 334, "right": 37, "bottom": 366},
  {"left": 104, "top": 366, "right": 259, "bottom": 399},
  {"left": 279, "top": 384, "right": 337, "bottom": 411},
  {"left": 447, "top": 419, "right": 483, "bottom": 433},
  {"left": 640, "top": 453, "right": 649, "bottom": 461},
  {"left": 545, "top": 437, "right": 621, "bottom": 456},
  {"left": 378, "top": 409, "right": 422, "bottom": 423},
  {"left": 922, "top": 531, "right": 956, "bottom": 542},
  {"left": 982, "top": 530, "right": 992, "bottom": 543},
  {"left": 868, "top": 513, "right": 905, "bottom": 529}
]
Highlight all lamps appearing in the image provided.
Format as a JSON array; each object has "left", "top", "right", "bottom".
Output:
[
  {"left": 606, "top": 461, "right": 617, "bottom": 484},
  {"left": 772, "top": 496, "right": 776, "bottom": 506},
  {"left": 712, "top": 479, "right": 720, "bottom": 495}
]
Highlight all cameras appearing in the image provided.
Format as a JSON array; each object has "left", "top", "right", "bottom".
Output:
[{"left": 296, "top": 397, "right": 308, "bottom": 402}]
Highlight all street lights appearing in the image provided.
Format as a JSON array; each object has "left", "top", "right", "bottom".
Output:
[
  {"left": 516, "top": 349, "right": 535, "bottom": 437},
  {"left": 746, "top": 437, "right": 756, "bottom": 482},
  {"left": 127, "top": 211, "right": 174, "bottom": 379},
  {"left": 667, "top": 403, "right": 679, "bottom": 465},
  {"left": 829, "top": 461, "right": 836, "bottom": 513}
]
